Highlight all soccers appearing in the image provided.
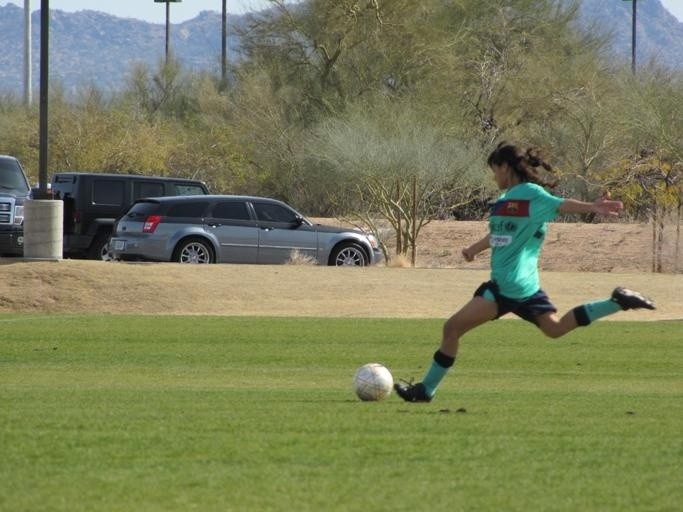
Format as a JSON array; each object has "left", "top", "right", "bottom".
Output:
[{"left": 352, "top": 362, "right": 393, "bottom": 401}]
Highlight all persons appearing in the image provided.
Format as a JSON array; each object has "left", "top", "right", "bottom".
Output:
[{"left": 393, "top": 142, "right": 657, "bottom": 404}]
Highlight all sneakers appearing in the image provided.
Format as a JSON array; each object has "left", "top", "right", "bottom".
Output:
[
  {"left": 394, "top": 383, "right": 436, "bottom": 403},
  {"left": 610, "top": 286, "right": 657, "bottom": 311}
]
